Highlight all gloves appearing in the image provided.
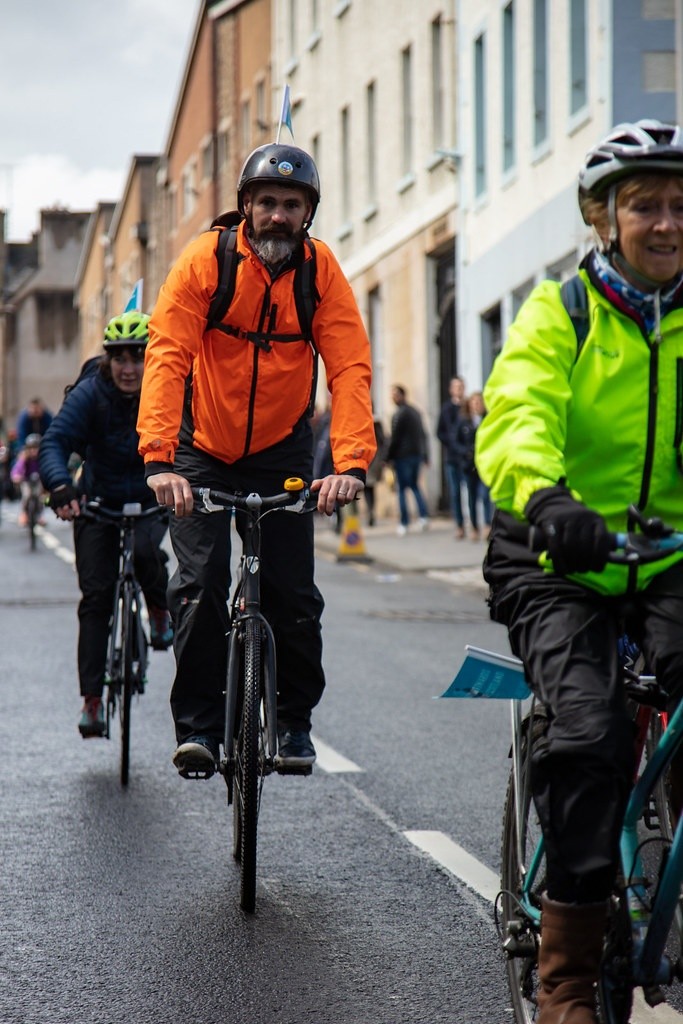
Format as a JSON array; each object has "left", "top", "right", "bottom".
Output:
[{"left": 523, "top": 476, "right": 609, "bottom": 575}]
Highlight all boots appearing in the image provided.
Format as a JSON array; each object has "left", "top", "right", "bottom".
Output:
[{"left": 536, "top": 890, "right": 611, "bottom": 1024}]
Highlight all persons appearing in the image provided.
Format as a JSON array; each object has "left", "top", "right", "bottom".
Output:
[
  {"left": 349, "top": 402, "right": 390, "bottom": 527},
  {"left": 437, "top": 377, "right": 494, "bottom": 538},
  {"left": 11, "top": 434, "right": 48, "bottom": 526},
  {"left": 483, "top": 120, "right": 683, "bottom": 1024},
  {"left": 15, "top": 398, "right": 52, "bottom": 442},
  {"left": 35, "top": 311, "right": 173, "bottom": 734},
  {"left": 134, "top": 143, "right": 378, "bottom": 766},
  {"left": 452, "top": 393, "right": 487, "bottom": 540},
  {"left": 0, "top": 427, "right": 19, "bottom": 501},
  {"left": 383, "top": 386, "right": 430, "bottom": 535}
]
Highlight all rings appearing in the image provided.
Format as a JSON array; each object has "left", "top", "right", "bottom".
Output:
[{"left": 338, "top": 491, "right": 346, "bottom": 495}]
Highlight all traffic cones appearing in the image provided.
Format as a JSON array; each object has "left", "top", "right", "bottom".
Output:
[{"left": 336, "top": 514, "right": 372, "bottom": 562}]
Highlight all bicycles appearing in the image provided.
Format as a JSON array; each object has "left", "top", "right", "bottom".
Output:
[
  {"left": 489, "top": 497, "right": 683, "bottom": 1024},
  {"left": 12, "top": 470, "right": 51, "bottom": 552},
  {"left": 54, "top": 485, "right": 174, "bottom": 788},
  {"left": 160, "top": 471, "right": 361, "bottom": 916}
]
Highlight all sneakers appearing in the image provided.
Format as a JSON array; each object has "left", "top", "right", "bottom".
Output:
[
  {"left": 78, "top": 694, "right": 104, "bottom": 735},
  {"left": 147, "top": 604, "right": 175, "bottom": 649},
  {"left": 171, "top": 732, "right": 221, "bottom": 767},
  {"left": 276, "top": 715, "right": 316, "bottom": 766}
]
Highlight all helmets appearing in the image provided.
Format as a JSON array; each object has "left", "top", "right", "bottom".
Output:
[
  {"left": 102, "top": 312, "right": 151, "bottom": 351},
  {"left": 237, "top": 142, "right": 320, "bottom": 223},
  {"left": 577, "top": 119, "right": 683, "bottom": 227}
]
